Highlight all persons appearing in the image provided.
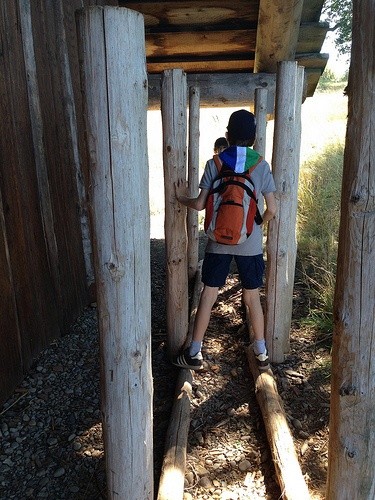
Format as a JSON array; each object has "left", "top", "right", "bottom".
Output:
[
  {"left": 214, "top": 137, "right": 228, "bottom": 155},
  {"left": 170, "top": 110, "right": 277, "bottom": 370}
]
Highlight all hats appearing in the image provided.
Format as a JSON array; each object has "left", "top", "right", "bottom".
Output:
[{"left": 225, "top": 110, "right": 256, "bottom": 134}]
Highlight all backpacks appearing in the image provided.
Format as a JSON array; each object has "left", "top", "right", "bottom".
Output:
[{"left": 203, "top": 153, "right": 262, "bottom": 245}]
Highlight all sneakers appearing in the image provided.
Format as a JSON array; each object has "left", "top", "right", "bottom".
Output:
[
  {"left": 171, "top": 350, "right": 204, "bottom": 370},
  {"left": 261, "top": 356, "right": 270, "bottom": 370}
]
